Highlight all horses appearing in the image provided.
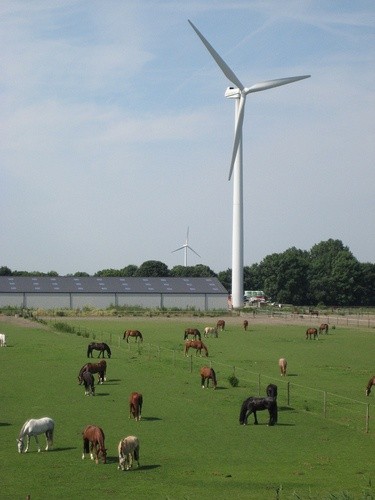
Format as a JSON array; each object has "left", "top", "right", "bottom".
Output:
[
  {"left": 237, "top": 395, "right": 278, "bottom": 428},
  {"left": 122, "top": 328, "right": 144, "bottom": 344},
  {"left": 319, "top": 323, "right": 329, "bottom": 335},
  {"left": 242, "top": 319, "right": 249, "bottom": 332},
  {"left": 364, "top": 375, "right": 375, "bottom": 397},
  {"left": 265, "top": 383, "right": 278, "bottom": 401},
  {"left": 183, "top": 328, "right": 202, "bottom": 341},
  {"left": 127, "top": 391, "right": 145, "bottom": 422},
  {"left": 203, "top": 326, "right": 219, "bottom": 339},
  {"left": 15, "top": 416, "right": 55, "bottom": 454},
  {"left": 308, "top": 308, "right": 319, "bottom": 318},
  {"left": 0, "top": 333, "right": 8, "bottom": 347},
  {"left": 278, "top": 357, "right": 288, "bottom": 377},
  {"left": 306, "top": 327, "right": 318, "bottom": 340},
  {"left": 117, "top": 435, "right": 142, "bottom": 471},
  {"left": 86, "top": 342, "right": 112, "bottom": 359},
  {"left": 216, "top": 319, "right": 225, "bottom": 332},
  {"left": 76, "top": 359, "right": 107, "bottom": 398},
  {"left": 81, "top": 423, "right": 109, "bottom": 465},
  {"left": 183, "top": 338, "right": 209, "bottom": 358},
  {"left": 199, "top": 366, "right": 219, "bottom": 391}
]
